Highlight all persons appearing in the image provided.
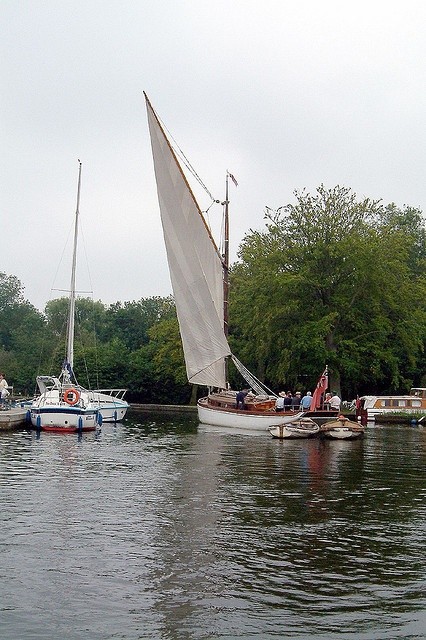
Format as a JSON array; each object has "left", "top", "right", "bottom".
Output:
[
  {"left": 0, "top": 373, "right": 10, "bottom": 411},
  {"left": 299, "top": 391, "right": 313, "bottom": 409},
  {"left": 275, "top": 391, "right": 302, "bottom": 412},
  {"left": 326, "top": 391, "right": 342, "bottom": 410}
]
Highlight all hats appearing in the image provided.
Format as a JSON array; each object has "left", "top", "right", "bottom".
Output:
[
  {"left": 307, "top": 391, "right": 311, "bottom": 395},
  {"left": 294, "top": 391, "right": 303, "bottom": 396},
  {"left": 279, "top": 391, "right": 286, "bottom": 397}
]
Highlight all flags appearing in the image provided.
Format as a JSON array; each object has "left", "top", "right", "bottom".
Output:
[{"left": 311, "top": 366, "right": 327, "bottom": 411}]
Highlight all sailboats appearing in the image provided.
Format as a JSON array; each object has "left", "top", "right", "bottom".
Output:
[
  {"left": 144, "top": 90, "right": 340, "bottom": 432},
  {"left": 29, "top": 163, "right": 130, "bottom": 432}
]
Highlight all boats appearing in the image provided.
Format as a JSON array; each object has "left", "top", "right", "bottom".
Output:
[
  {"left": 0, "top": 409, "right": 27, "bottom": 430},
  {"left": 15, "top": 374, "right": 125, "bottom": 422},
  {"left": 320, "top": 418, "right": 364, "bottom": 439},
  {"left": 350, "top": 388, "right": 426, "bottom": 420},
  {"left": 268, "top": 418, "right": 319, "bottom": 437}
]
